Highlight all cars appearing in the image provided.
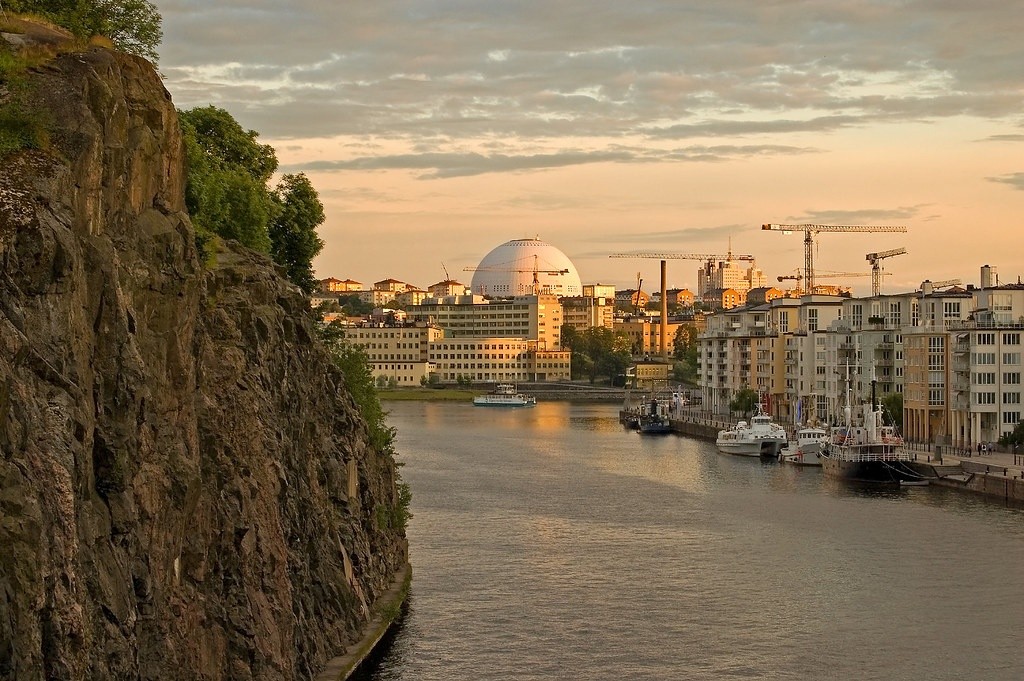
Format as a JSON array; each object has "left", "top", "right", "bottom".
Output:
[{"left": 359, "top": 318, "right": 368, "bottom": 323}]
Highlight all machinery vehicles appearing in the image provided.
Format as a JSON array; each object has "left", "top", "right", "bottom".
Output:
[
  {"left": 440, "top": 260, "right": 451, "bottom": 281},
  {"left": 917, "top": 278, "right": 963, "bottom": 291},
  {"left": 759, "top": 222, "right": 909, "bottom": 295}
]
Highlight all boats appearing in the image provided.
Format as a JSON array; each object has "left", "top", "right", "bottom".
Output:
[
  {"left": 625, "top": 415, "right": 639, "bottom": 424},
  {"left": 637, "top": 398, "right": 672, "bottom": 432},
  {"left": 473, "top": 380, "right": 537, "bottom": 407},
  {"left": 715, "top": 389, "right": 788, "bottom": 457},
  {"left": 780, "top": 418, "right": 831, "bottom": 465},
  {"left": 818, "top": 357, "right": 916, "bottom": 484}
]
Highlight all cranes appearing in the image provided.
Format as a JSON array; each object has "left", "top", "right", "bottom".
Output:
[
  {"left": 607, "top": 251, "right": 754, "bottom": 268},
  {"left": 461, "top": 254, "right": 569, "bottom": 286},
  {"left": 866, "top": 247, "right": 907, "bottom": 297},
  {"left": 776, "top": 268, "right": 892, "bottom": 283}
]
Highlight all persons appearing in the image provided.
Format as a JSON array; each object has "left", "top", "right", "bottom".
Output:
[
  {"left": 978, "top": 441, "right": 993, "bottom": 456},
  {"left": 1014, "top": 442, "right": 1018, "bottom": 454},
  {"left": 967, "top": 443, "right": 972, "bottom": 457}
]
